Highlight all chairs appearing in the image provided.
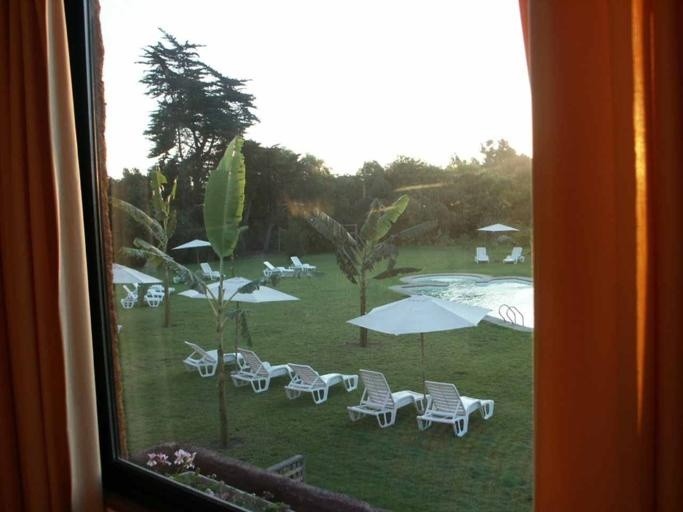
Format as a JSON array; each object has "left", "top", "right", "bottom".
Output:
[
  {"left": 200, "top": 262, "right": 226, "bottom": 281},
  {"left": 284, "top": 364, "right": 359, "bottom": 404},
  {"left": 263, "top": 256, "right": 317, "bottom": 278},
  {"left": 183, "top": 340, "right": 246, "bottom": 378},
  {"left": 474, "top": 247, "right": 489, "bottom": 264},
  {"left": 416, "top": 380, "right": 496, "bottom": 438},
  {"left": 230, "top": 348, "right": 294, "bottom": 393},
  {"left": 346, "top": 368, "right": 431, "bottom": 428},
  {"left": 120, "top": 284, "right": 176, "bottom": 309},
  {"left": 502, "top": 246, "right": 526, "bottom": 264}
]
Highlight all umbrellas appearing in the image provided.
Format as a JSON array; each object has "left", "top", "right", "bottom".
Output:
[
  {"left": 345, "top": 292, "right": 492, "bottom": 405},
  {"left": 176, "top": 272, "right": 303, "bottom": 373},
  {"left": 112, "top": 264, "right": 164, "bottom": 285},
  {"left": 171, "top": 237, "right": 214, "bottom": 265},
  {"left": 475, "top": 221, "right": 520, "bottom": 259}
]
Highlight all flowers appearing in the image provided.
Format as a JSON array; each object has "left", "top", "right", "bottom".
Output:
[{"left": 146, "top": 449, "right": 291, "bottom": 512}]
